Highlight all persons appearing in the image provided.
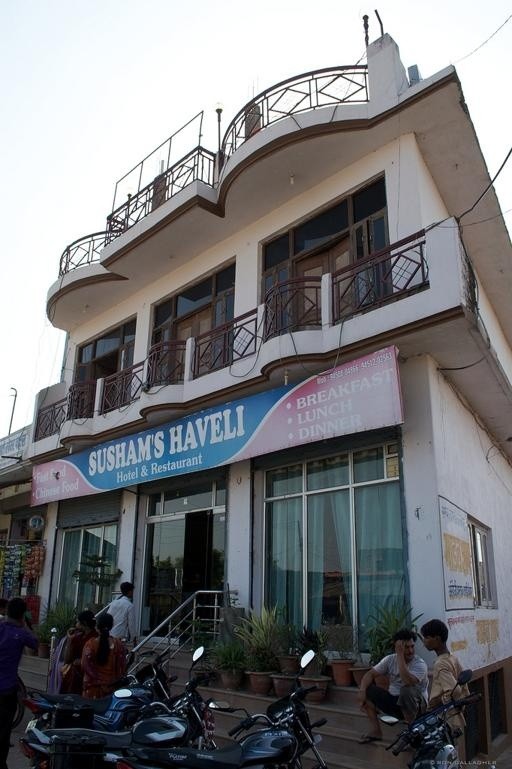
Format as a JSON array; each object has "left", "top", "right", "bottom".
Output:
[
  {"left": 421, "top": 618, "right": 470, "bottom": 767},
  {"left": 355, "top": 629, "right": 432, "bottom": 745},
  {"left": 0, "top": 581, "right": 138, "bottom": 769}
]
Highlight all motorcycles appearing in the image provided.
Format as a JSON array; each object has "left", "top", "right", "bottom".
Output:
[
  {"left": 378, "top": 668, "right": 485, "bottom": 769},
  {"left": 17, "top": 637, "right": 177, "bottom": 736},
  {"left": 97, "top": 648, "right": 328, "bottom": 769},
  {"left": 19, "top": 644, "right": 220, "bottom": 768},
  {"left": 11, "top": 674, "right": 27, "bottom": 730}
]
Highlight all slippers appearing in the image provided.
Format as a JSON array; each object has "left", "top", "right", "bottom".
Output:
[{"left": 356, "top": 735, "right": 383, "bottom": 744}]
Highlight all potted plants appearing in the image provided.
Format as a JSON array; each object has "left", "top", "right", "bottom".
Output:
[
  {"left": 23, "top": 598, "right": 83, "bottom": 659},
  {"left": 203, "top": 601, "right": 418, "bottom": 708}
]
